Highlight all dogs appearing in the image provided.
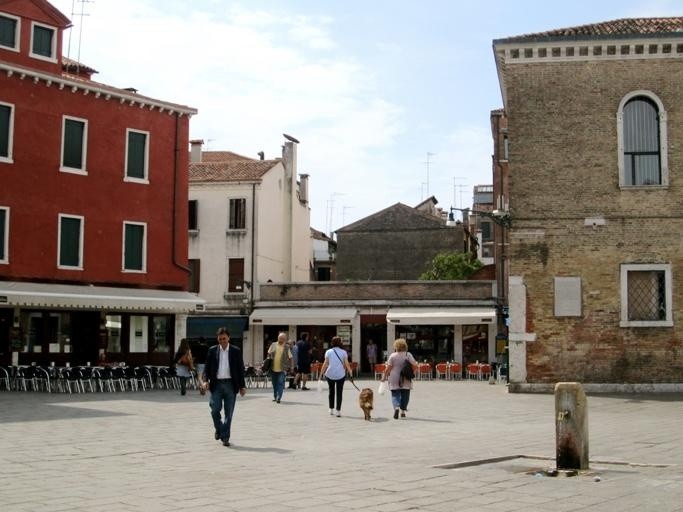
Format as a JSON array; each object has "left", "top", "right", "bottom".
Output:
[{"left": 358, "top": 388, "right": 374, "bottom": 421}]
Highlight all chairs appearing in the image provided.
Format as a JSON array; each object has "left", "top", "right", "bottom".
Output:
[
  {"left": 308, "top": 363, "right": 508, "bottom": 381},
  {"left": 0, "top": 364, "right": 295, "bottom": 394}
]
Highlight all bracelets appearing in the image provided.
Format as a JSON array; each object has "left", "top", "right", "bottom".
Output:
[
  {"left": 382, "top": 373, "right": 386, "bottom": 379},
  {"left": 289, "top": 367, "right": 294, "bottom": 370}
]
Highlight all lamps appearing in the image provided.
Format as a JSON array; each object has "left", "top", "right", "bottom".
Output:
[
  {"left": 236, "top": 279, "right": 252, "bottom": 290},
  {"left": 445, "top": 206, "right": 510, "bottom": 231}
]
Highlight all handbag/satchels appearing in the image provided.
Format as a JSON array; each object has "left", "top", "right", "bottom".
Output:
[
  {"left": 181, "top": 348, "right": 191, "bottom": 366},
  {"left": 397, "top": 351, "right": 416, "bottom": 388},
  {"left": 260, "top": 352, "right": 273, "bottom": 373}
]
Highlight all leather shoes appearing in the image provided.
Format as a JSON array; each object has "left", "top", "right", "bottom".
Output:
[
  {"left": 291, "top": 384, "right": 310, "bottom": 390},
  {"left": 220, "top": 435, "right": 231, "bottom": 446},
  {"left": 215, "top": 429, "right": 220, "bottom": 440}
]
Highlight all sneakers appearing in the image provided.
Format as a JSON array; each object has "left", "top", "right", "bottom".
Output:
[
  {"left": 393, "top": 408, "right": 406, "bottom": 419},
  {"left": 272, "top": 396, "right": 281, "bottom": 403},
  {"left": 327, "top": 407, "right": 342, "bottom": 417}
]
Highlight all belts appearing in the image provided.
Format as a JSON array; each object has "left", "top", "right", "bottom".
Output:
[{"left": 217, "top": 378, "right": 232, "bottom": 383}]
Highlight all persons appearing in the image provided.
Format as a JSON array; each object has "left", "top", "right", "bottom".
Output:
[
  {"left": 265, "top": 331, "right": 294, "bottom": 403},
  {"left": 366, "top": 338, "right": 378, "bottom": 375},
  {"left": 318, "top": 336, "right": 352, "bottom": 417},
  {"left": 380, "top": 338, "right": 417, "bottom": 419},
  {"left": 286, "top": 339, "right": 298, "bottom": 388},
  {"left": 201, "top": 326, "right": 245, "bottom": 445},
  {"left": 173, "top": 338, "right": 193, "bottom": 396},
  {"left": 193, "top": 335, "right": 207, "bottom": 396},
  {"left": 291, "top": 331, "right": 312, "bottom": 390}
]
